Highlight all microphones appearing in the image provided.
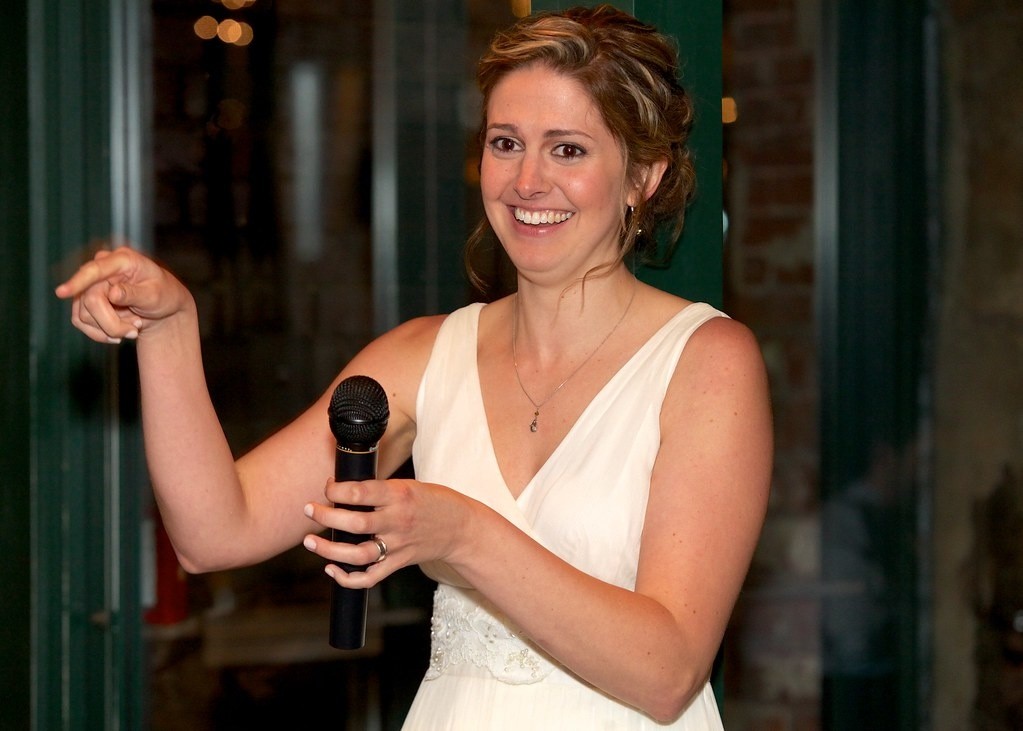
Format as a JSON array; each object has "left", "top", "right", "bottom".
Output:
[{"left": 326, "top": 374, "right": 391, "bottom": 651}]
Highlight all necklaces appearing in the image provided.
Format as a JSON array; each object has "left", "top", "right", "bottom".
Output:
[{"left": 512, "top": 274, "right": 637, "bottom": 432}]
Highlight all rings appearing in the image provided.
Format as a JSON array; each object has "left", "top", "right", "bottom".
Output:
[{"left": 369, "top": 537, "right": 388, "bottom": 562}]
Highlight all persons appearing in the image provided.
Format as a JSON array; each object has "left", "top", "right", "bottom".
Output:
[{"left": 56, "top": 1, "right": 775, "bottom": 731}]
